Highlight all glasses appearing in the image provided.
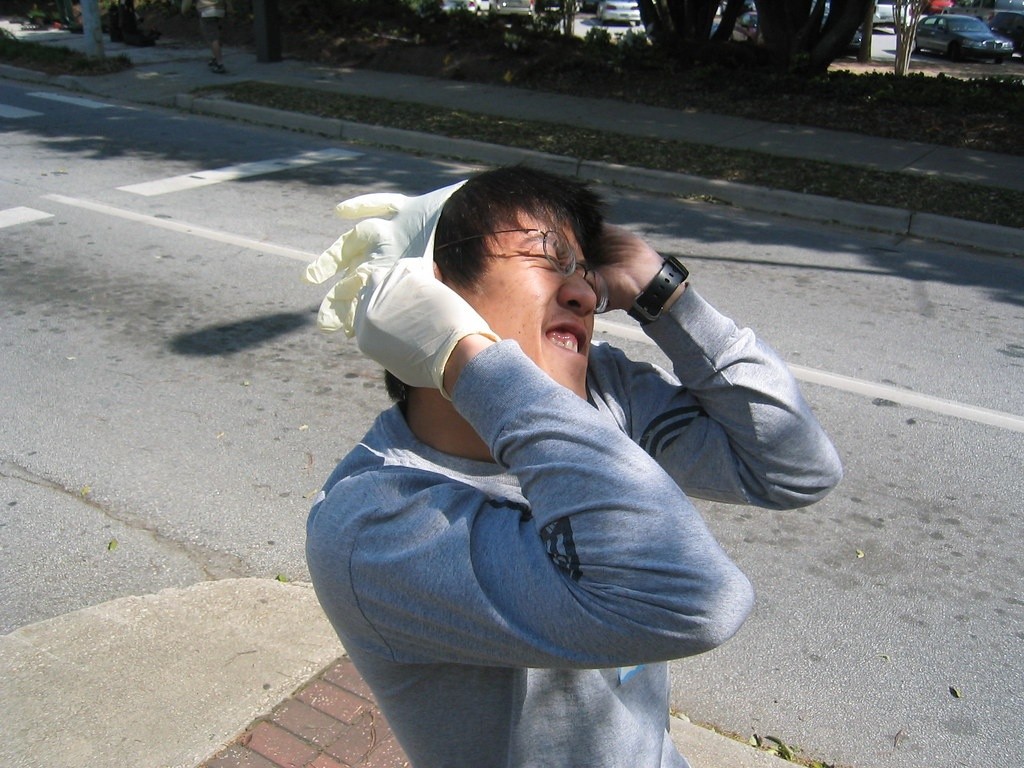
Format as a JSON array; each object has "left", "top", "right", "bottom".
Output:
[{"left": 433, "top": 226, "right": 610, "bottom": 315}]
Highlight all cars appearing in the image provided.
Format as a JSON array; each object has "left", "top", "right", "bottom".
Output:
[
  {"left": 596, "top": 0, "right": 641, "bottom": 26},
  {"left": 810, "top": 0, "right": 862, "bottom": 55},
  {"left": 467, "top": 0, "right": 490, "bottom": 16},
  {"left": 740, "top": 12, "right": 757, "bottom": 33},
  {"left": 535, "top": 0, "right": 564, "bottom": 14},
  {"left": 872, "top": 0, "right": 928, "bottom": 34},
  {"left": 708, "top": 23, "right": 733, "bottom": 41},
  {"left": 911, "top": 14, "right": 1016, "bottom": 64},
  {"left": 489, "top": 0, "right": 535, "bottom": 17},
  {"left": 982, "top": 9, "right": 1024, "bottom": 60}
]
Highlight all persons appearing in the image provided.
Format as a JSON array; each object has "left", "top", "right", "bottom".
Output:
[
  {"left": 305, "top": 164, "right": 844, "bottom": 768},
  {"left": 196, "top": 0, "right": 227, "bottom": 73}
]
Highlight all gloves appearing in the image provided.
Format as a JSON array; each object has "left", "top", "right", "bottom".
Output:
[
  {"left": 304, "top": 179, "right": 468, "bottom": 338},
  {"left": 353, "top": 255, "right": 503, "bottom": 402}
]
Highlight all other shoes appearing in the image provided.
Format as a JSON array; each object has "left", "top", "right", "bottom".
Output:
[{"left": 206, "top": 57, "right": 225, "bottom": 74}]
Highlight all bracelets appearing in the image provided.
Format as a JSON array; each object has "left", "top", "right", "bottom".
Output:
[{"left": 627, "top": 255, "right": 689, "bottom": 325}]
